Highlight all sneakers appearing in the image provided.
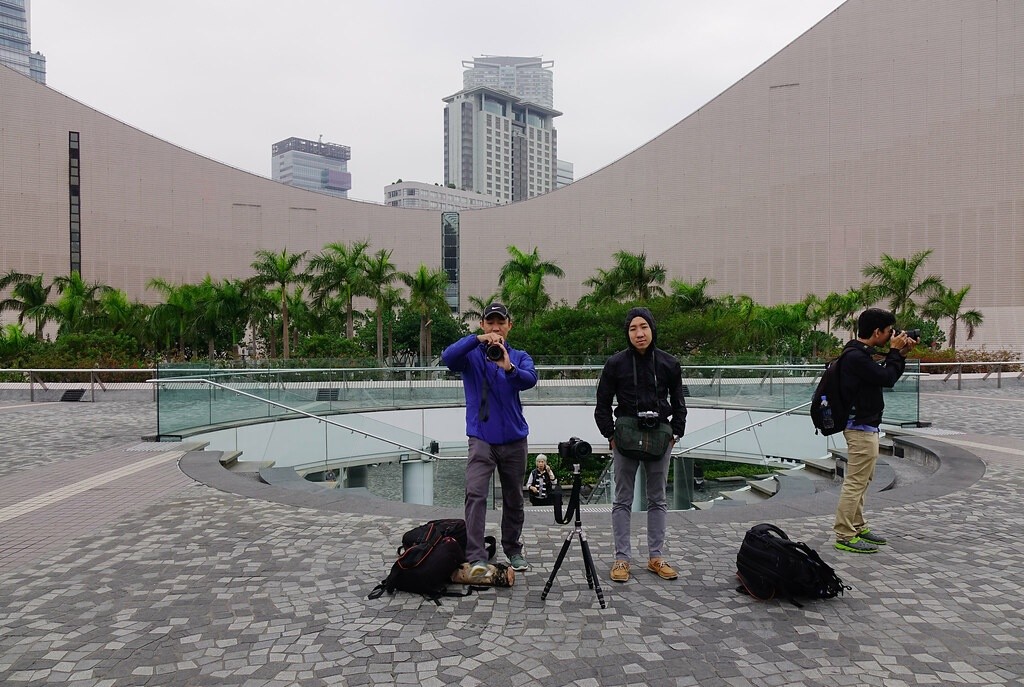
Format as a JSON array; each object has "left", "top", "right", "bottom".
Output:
[
  {"left": 856, "top": 528, "right": 887, "bottom": 544},
  {"left": 647, "top": 557, "right": 677, "bottom": 579},
  {"left": 506, "top": 553, "right": 528, "bottom": 571},
  {"left": 610, "top": 560, "right": 631, "bottom": 581},
  {"left": 835, "top": 536, "right": 878, "bottom": 553},
  {"left": 468, "top": 560, "right": 487, "bottom": 576}
]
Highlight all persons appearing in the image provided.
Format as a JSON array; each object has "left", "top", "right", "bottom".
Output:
[
  {"left": 441, "top": 303, "right": 537, "bottom": 581},
  {"left": 527, "top": 453, "right": 557, "bottom": 506},
  {"left": 832, "top": 308, "right": 921, "bottom": 553},
  {"left": 595, "top": 308, "right": 687, "bottom": 583}
]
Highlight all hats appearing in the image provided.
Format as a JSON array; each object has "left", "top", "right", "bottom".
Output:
[{"left": 482, "top": 303, "right": 510, "bottom": 319}]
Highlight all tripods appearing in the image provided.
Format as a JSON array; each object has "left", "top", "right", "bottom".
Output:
[{"left": 541, "top": 461, "right": 606, "bottom": 609}]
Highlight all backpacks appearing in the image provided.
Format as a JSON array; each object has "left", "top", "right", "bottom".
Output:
[
  {"left": 810, "top": 348, "right": 869, "bottom": 437},
  {"left": 368, "top": 519, "right": 496, "bottom": 606},
  {"left": 736, "top": 524, "right": 852, "bottom": 608}
]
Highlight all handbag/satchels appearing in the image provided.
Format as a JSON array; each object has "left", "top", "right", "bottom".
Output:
[{"left": 615, "top": 416, "right": 673, "bottom": 461}]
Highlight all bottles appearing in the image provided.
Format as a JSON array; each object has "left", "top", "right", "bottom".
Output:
[{"left": 820, "top": 396, "right": 834, "bottom": 430}]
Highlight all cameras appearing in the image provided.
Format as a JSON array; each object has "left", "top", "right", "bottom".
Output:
[
  {"left": 479, "top": 340, "right": 504, "bottom": 361},
  {"left": 557, "top": 437, "right": 593, "bottom": 460},
  {"left": 891, "top": 328, "right": 920, "bottom": 341}
]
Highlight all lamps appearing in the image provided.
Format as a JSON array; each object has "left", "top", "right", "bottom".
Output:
[
  {"left": 399, "top": 454, "right": 409, "bottom": 461},
  {"left": 430, "top": 439, "right": 439, "bottom": 456}
]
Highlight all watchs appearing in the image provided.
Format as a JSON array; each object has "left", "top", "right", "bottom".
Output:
[{"left": 505, "top": 362, "right": 516, "bottom": 374}]
